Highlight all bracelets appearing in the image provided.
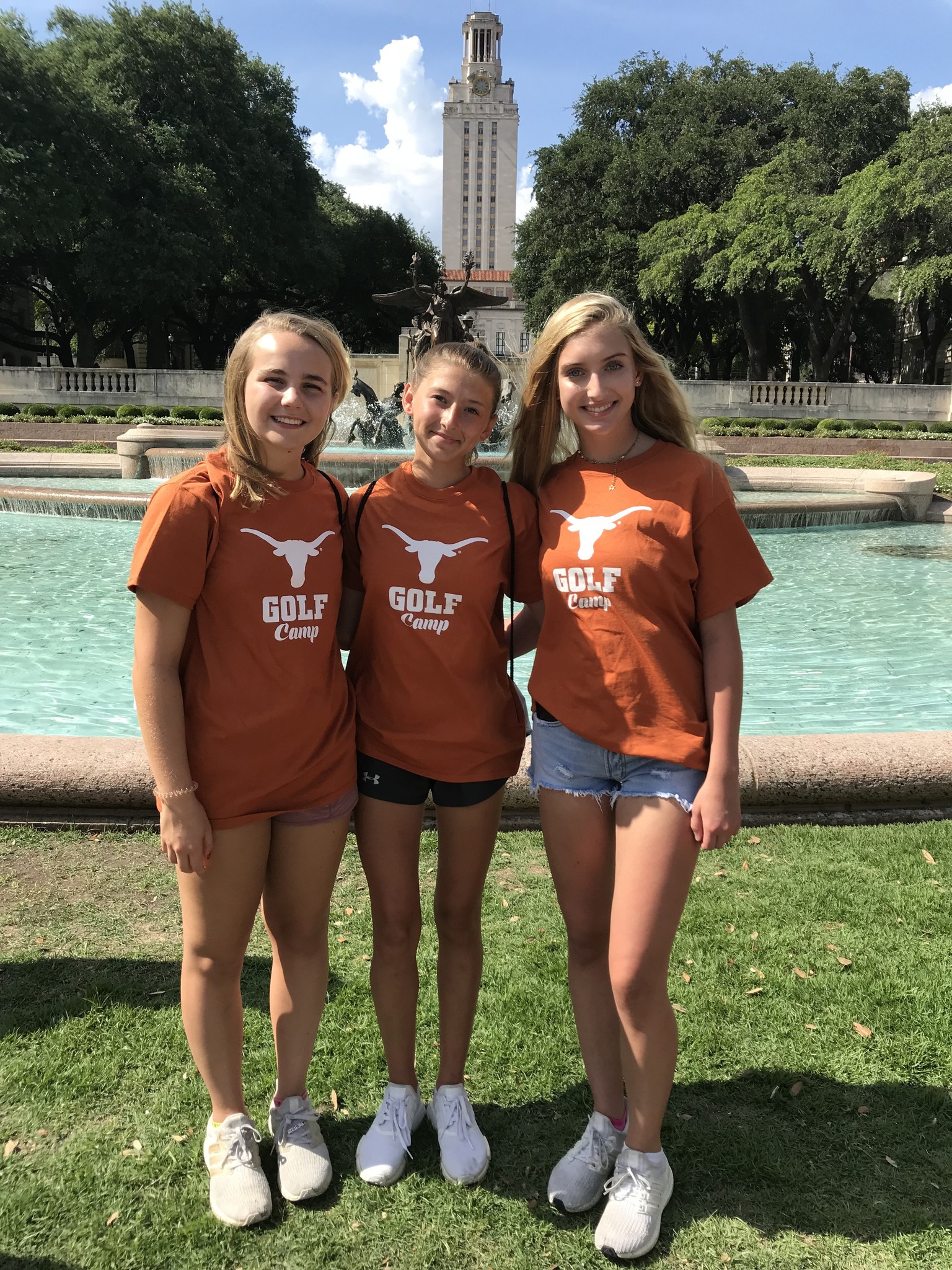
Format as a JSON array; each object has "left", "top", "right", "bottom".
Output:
[{"left": 152, "top": 782, "right": 198, "bottom": 798}]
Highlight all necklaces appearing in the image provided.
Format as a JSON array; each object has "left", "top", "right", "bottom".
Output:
[{"left": 577, "top": 431, "right": 640, "bottom": 489}]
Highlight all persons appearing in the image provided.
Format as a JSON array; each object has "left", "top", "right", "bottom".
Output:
[
  {"left": 342, "top": 341, "right": 536, "bottom": 1183},
  {"left": 123, "top": 312, "right": 358, "bottom": 1232},
  {"left": 380, "top": 381, "right": 404, "bottom": 418},
  {"left": 372, "top": 261, "right": 508, "bottom": 366},
  {"left": 502, "top": 289, "right": 773, "bottom": 1258}
]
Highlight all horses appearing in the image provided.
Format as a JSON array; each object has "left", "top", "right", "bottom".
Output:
[{"left": 346, "top": 369, "right": 397, "bottom": 448}]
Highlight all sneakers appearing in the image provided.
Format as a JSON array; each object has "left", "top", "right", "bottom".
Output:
[
  {"left": 268, "top": 1077, "right": 332, "bottom": 1202},
  {"left": 548, "top": 1094, "right": 631, "bottom": 1215},
  {"left": 426, "top": 1082, "right": 491, "bottom": 1187},
  {"left": 356, "top": 1083, "right": 425, "bottom": 1185},
  {"left": 203, "top": 1110, "right": 272, "bottom": 1226},
  {"left": 595, "top": 1148, "right": 673, "bottom": 1260}
]
